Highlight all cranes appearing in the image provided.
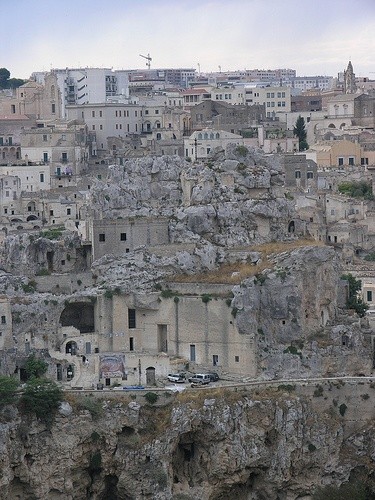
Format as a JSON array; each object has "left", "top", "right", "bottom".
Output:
[{"left": 138, "top": 52, "right": 152, "bottom": 69}]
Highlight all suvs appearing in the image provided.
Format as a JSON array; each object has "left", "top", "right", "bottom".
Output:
[
  {"left": 188, "top": 373, "right": 210, "bottom": 385},
  {"left": 168, "top": 373, "right": 186, "bottom": 383},
  {"left": 208, "top": 373, "right": 219, "bottom": 382}
]
